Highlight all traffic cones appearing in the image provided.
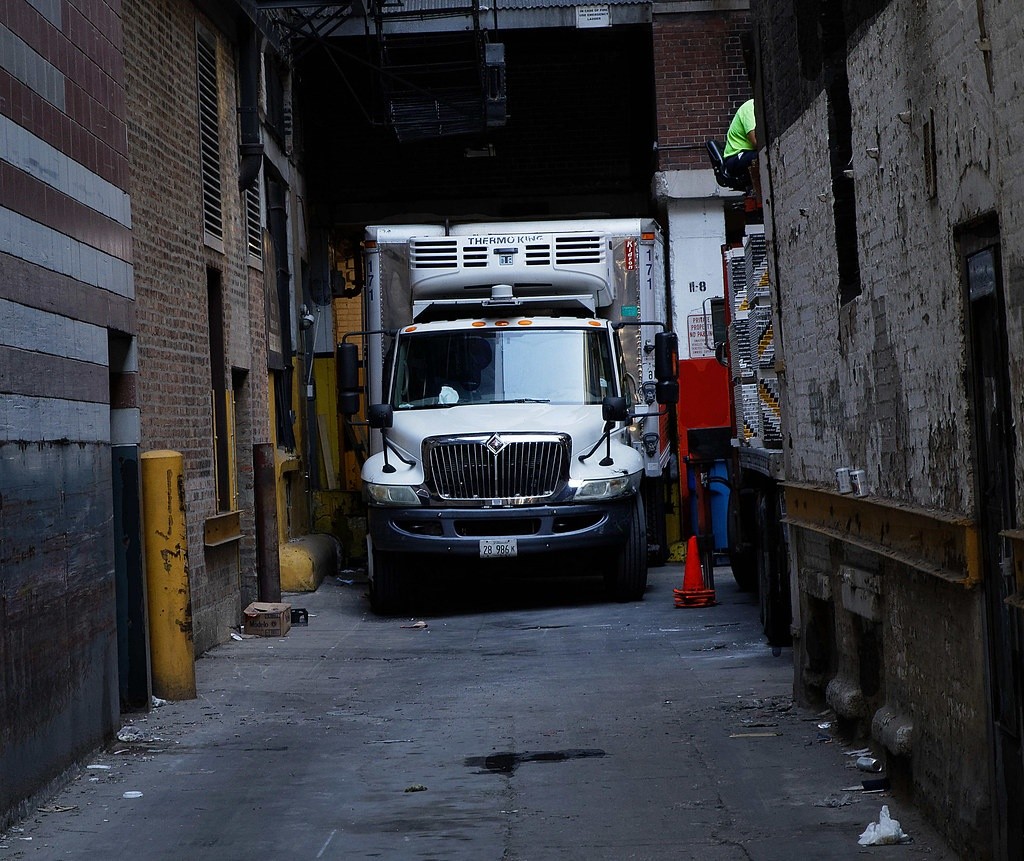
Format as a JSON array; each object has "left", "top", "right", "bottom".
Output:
[{"left": 676, "top": 537, "right": 715, "bottom": 608}]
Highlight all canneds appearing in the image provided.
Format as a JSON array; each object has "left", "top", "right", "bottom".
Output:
[
  {"left": 835, "top": 466, "right": 853, "bottom": 494},
  {"left": 849, "top": 468, "right": 869, "bottom": 498}
]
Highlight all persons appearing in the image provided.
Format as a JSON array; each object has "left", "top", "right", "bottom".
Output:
[{"left": 723, "top": 99, "right": 757, "bottom": 179}]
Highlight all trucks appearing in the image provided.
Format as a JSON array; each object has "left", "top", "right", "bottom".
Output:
[{"left": 337, "top": 219, "right": 683, "bottom": 616}]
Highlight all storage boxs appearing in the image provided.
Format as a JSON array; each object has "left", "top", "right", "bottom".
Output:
[{"left": 244, "top": 601, "right": 291, "bottom": 636}]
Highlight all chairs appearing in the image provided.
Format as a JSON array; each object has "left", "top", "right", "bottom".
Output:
[
  {"left": 705, "top": 140, "right": 762, "bottom": 211},
  {"left": 426, "top": 363, "right": 481, "bottom": 405}
]
